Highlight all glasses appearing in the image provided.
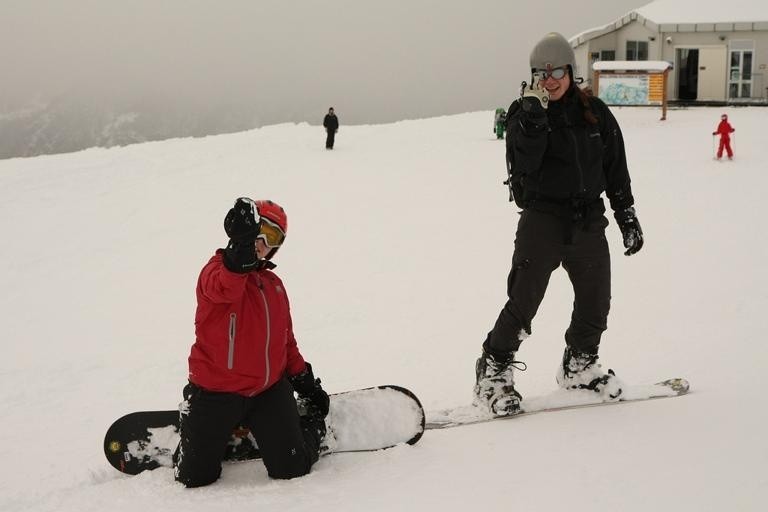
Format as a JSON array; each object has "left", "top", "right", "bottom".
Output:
[
  {"left": 531, "top": 68, "right": 569, "bottom": 80},
  {"left": 257, "top": 217, "right": 285, "bottom": 246}
]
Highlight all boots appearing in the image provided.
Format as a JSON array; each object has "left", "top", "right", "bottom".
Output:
[
  {"left": 474, "top": 343, "right": 521, "bottom": 415},
  {"left": 556, "top": 346, "right": 620, "bottom": 398}
]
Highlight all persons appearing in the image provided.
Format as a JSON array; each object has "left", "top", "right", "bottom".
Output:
[
  {"left": 494, "top": 108, "right": 507, "bottom": 139},
  {"left": 476, "top": 33, "right": 644, "bottom": 414},
  {"left": 323, "top": 107, "right": 338, "bottom": 149},
  {"left": 173, "top": 198, "right": 330, "bottom": 488},
  {"left": 713, "top": 114, "right": 735, "bottom": 159}
]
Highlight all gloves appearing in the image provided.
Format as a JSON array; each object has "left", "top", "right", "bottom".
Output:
[
  {"left": 291, "top": 363, "right": 328, "bottom": 420},
  {"left": 615, "top": 205, "right": 643, "bottom": 257},
  {"left": 222, "top": 200, "right": 260, "bottom": 272},
  {"left": 519, "top": 96, "right": 551, "bottom": 134}
]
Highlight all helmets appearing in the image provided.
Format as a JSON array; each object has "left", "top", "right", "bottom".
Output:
[
  {"left": 254, "top": 199, "right": 287, "bottom": 248},
  {"left": 529, "top": 31, "right": 578, "bottom": 87}
]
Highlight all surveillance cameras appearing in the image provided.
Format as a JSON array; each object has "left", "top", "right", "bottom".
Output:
[
  {"left": 647, "top": 36, "right": 655, "bottom": 41},
  {"left": 665, "top": 37, "right": 673, "bottom": 44}
]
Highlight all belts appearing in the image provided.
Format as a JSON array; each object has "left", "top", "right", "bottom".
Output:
[{"left": 533, "top": 199, "right": 604, "bottom": 215}]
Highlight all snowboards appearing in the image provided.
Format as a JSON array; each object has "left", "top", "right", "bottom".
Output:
[
  {"left": 104, "top": 385, "right": 426, "bottom": 475},
  {"left": 424, "top": 378, "right": 689, "bottom": 430}
]
